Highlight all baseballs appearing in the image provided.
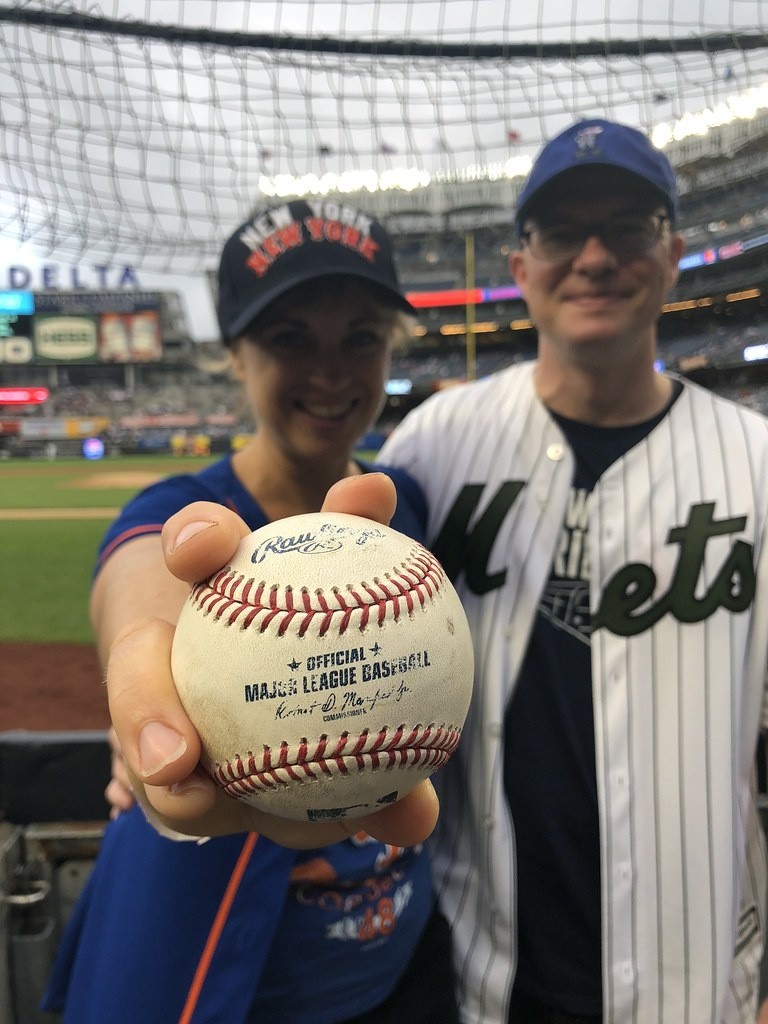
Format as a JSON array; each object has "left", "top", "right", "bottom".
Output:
[{"left": 171, "top": 511, "right": 479, "bottom": 821}]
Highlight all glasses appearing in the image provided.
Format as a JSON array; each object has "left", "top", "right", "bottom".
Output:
[{"left": 522, "top": 211, "right": 672, "bottom": 262}]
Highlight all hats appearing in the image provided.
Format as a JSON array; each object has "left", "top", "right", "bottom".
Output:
[
  {"left": 513, "top": 119, "right": 678, "bottom": 235},
  {"left": 217, "top": 200, "right": 418, "bottom": 338}
]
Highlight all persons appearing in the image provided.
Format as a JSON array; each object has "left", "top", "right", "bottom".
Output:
[
  {"left": 41, "top": 200, "right": 467, "bottom": 1024},
  {"left": 381, "top": 116, "right": 768, "bottom": 1024}
]
